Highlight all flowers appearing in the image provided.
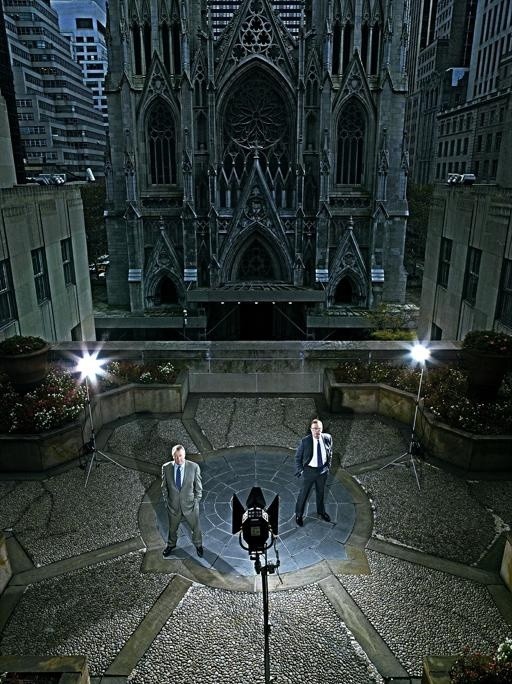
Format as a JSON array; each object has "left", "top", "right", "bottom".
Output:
[{"left": 448, "top": 645, "right": 505, "bottom": 684}]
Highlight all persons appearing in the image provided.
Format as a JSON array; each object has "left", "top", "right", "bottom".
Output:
[
  {"left": 293, "top": 416, "right": 334, "bottom": 527},
  {"left": 159, "top": 443, "right": 206, "bottom": 559}
]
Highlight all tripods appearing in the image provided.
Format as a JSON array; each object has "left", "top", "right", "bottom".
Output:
[
  {"left": 378, "top": 364, "right": 440, "bottom": 492},
  {"left": 84, "top": 377, "right": 127, "bottom": 489}
]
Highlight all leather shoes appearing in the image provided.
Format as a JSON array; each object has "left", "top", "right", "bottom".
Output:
[
  {"left": 196, "top": 546, "right": 203, "bottom": 557},
  {"left": 163, "top": 545, "right": 177, "bottom": 555},
  {"left": 318, "top": 511, "right": 330, "bottom": 521},
  {"left": 296, "top": 515, "right": 303, "bottom": 526}
]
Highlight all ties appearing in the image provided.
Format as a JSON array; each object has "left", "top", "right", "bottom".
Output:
[
  {"left": 176, "top": 465, "right": 182, "bottom": 488},
  {"left": 317, "top": 439, "right": 323, "bottom": 470}
]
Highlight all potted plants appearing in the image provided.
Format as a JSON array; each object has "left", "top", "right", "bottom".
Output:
[
  {"left": 459, "top": 329, "right": 512, "bottom": 381},
  {"left": 1, "top": 335, "right": 49, "bottom": 385}
]
